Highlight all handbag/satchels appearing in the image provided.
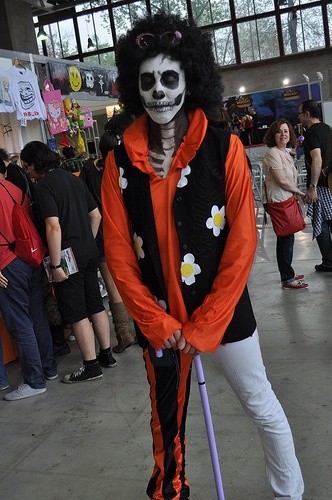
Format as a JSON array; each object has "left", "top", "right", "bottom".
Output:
[{"left": 263, "top": 194, "right": 306, "bottom": 238}]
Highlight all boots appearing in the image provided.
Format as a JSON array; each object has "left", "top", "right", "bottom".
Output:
[{"left": 108, "top": 301, "right": 134, "bottom": 353}]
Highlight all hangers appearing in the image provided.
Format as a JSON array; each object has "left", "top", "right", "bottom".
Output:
[{"left": 13, "top": 56, "right": 25, "bottom": 69}]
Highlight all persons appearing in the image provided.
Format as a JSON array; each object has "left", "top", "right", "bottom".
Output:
[
  {"left": 100, "top": 15, "right": 304, "bottom": 500},
  {"left": 262, "top": 119, "right": 308, "bottom": 291},
  {"left": 0, "top": 141, "right": 135, "bottom": 401},
  {"left": 297, "top": 99, "right": 332, "bottom": 272},
  {"left": 228, "top": 109, "right": 259, "bottom": 146}
]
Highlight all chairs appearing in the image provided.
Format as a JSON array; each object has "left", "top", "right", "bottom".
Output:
[{"left": 250, "top": 158, "right": 308, "bottom": 229}]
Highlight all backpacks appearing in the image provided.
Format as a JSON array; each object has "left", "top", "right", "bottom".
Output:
[{"left": 0, "top": 182, "right": 46, "bottom": 268}]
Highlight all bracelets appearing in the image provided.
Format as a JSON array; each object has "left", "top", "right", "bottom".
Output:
[{"left": 309, "top": 184, "right": 316, "bottom": 188}]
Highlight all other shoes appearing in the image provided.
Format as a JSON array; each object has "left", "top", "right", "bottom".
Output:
[
  {"left": 54, "top": 344, "right": 71, "bottom": 357},
  {"left": 281, "top": 275, "right": 304, "bottom": 284},
  {"left": 282, "top": 280, "right": 309, "bottom": 290},
  {"left": 315, "top": 264, "right": 332, "bottom": 272}
]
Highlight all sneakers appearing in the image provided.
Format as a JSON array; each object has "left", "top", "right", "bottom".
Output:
[
  {"left": 95, "top": 355, "right": 117, "bottom": 367},
  {"left": 62, "top": 363, "right": 103, "bottom": 384},
  {"left": 4, "top": 383, "right": 47, "bottom": 401},
  {"left": 44, "top": 374, "right": 58, "bottom": 379}
]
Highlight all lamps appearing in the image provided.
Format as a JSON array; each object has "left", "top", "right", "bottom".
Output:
[
  {"left": 37, "top": 22, "right": 48, "bottom": 40},
  {"left": 85, "top": 13, "right": 94, "bottom": 50}
]
